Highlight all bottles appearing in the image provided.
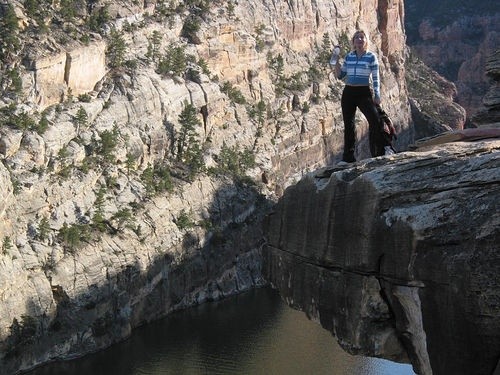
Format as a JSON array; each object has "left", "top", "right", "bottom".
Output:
[{"left": 330, "top": 44, "right": 341, "bottom": 65}]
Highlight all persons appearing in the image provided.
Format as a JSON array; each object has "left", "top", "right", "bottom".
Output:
[{"left": 332, "top": 29, "right": 386, "bottom": 166}]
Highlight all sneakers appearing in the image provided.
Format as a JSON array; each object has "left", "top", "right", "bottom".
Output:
[{"left": 337, "top": 160, "right": 353, "bottom": 166}]
